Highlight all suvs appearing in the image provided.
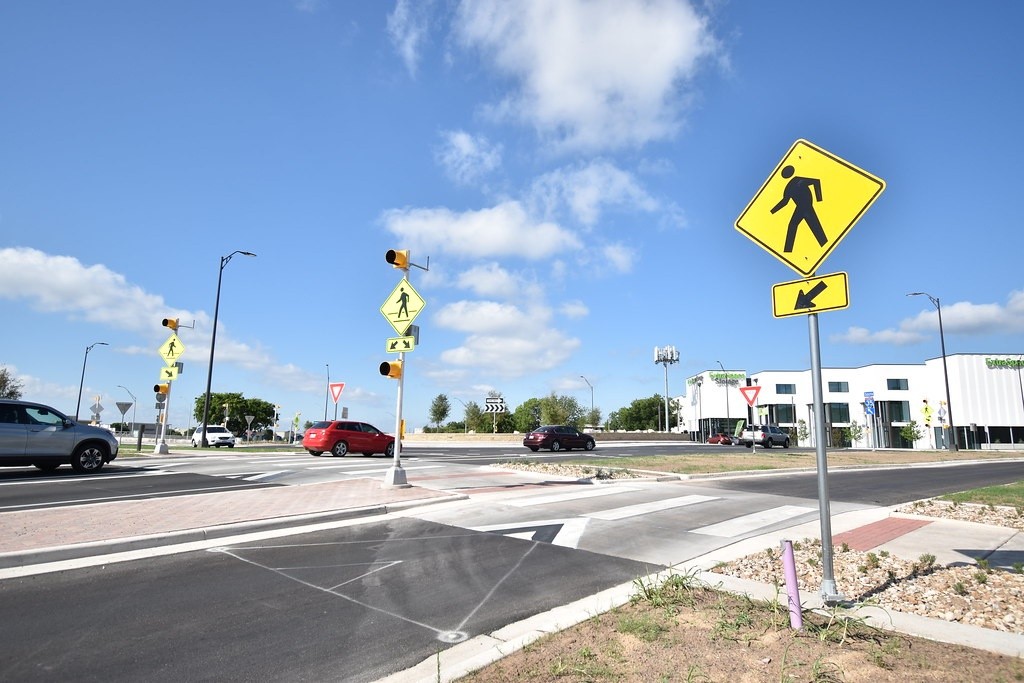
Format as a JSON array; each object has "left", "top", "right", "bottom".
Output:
[
  {"left": 0, "top": 399, "right": 119, "bottom": 475},
  {"left": 739, "top": 423, "right": 790, "bottom": 448}
]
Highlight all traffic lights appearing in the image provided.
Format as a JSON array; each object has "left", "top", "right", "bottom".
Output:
[
  {"left": 379, "top": 361, "right": 403, "bottom": 379},
  {"left": 385, "top": 249, "right": 410, "bottom": 270},
  {"left": 162, "top": 317, "right": 177, "bottom": 330},
  {"left": 222, "top": 403, "right": 227, "bottom": 408},
  {"left": 153, "top": 383, "right": 169, "bottom": 394}
]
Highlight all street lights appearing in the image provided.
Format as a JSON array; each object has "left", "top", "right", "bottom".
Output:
[
  {"left": 905, "top": 291, "right": 958, "bottom": 451},
  {"left": 716, "top": 360, "right": 730, "bottom": 437},
  {"left": 197, "top": 249, "right": 257, "bottom": 449},
  {"left": 76, "top": 341, "right": 109, "bottom": 422},
  {"left": 581, "top": 376, "right": 594, "bottom": 412},
  {"left": 118, "top": 386, "right": 136, "bottom": 437}
]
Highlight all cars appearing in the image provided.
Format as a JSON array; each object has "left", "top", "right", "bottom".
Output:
[
  {"left": 192, "top": 424, "right": 235, "bottom": 448},
  {"left": 301, "top": 420, "right": 402, "bottom": 458},
  {"left": 706, "top": 433, "right": 744, "bottom": 445},
  {"left": 523, "top": 425, "right": 596, "bottom": 452}
]
